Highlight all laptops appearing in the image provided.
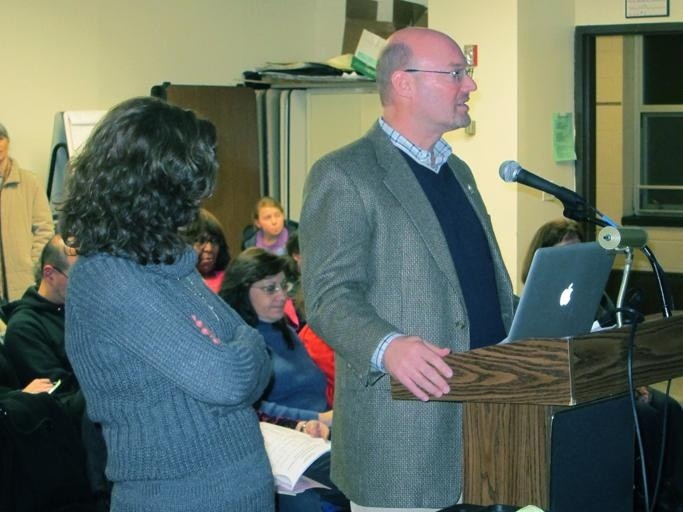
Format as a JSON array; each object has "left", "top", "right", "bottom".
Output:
[{"left": 493, "top": 241, "right": 617, "bottom": 346}]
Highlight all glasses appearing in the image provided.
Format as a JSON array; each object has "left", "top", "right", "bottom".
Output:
[
  {"left": 241, "top": 281, "right": 294, "bottom": 294},
  {"left": 49, "top": 263, "right": 69, "bottom": 281},
  {"left": 402, "top": 66, "right": 473, "bottom": 81},
  {"left": 191, "top": 236, "right": 218, "bottom": 244}
]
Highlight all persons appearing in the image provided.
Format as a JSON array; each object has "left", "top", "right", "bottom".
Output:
[
  {"left": 519, "top": 216, "right": 683, "bottom": 511},
  {"left": 300, "top": 26, "right": 517, "bottom": 512},
  {"left": 62, "top": 98, "right": 278, "bottom": 512},
  {"left": 0, "top": 124, "right": 54, "bottom": 342},
  {"left": 190, "top": 194, "right": 353, "bottom": 511},
  {"left": 1, "top": 193, "right": 113, "bottom": 510}
]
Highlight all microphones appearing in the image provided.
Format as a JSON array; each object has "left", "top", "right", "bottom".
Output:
[{"left": 499, "top": 160, "right": 589, "bottom": 222}]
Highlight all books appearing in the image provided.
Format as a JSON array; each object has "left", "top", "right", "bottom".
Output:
[{"left": 263, "top": 28, "right": 389, "bottom": 78}]
